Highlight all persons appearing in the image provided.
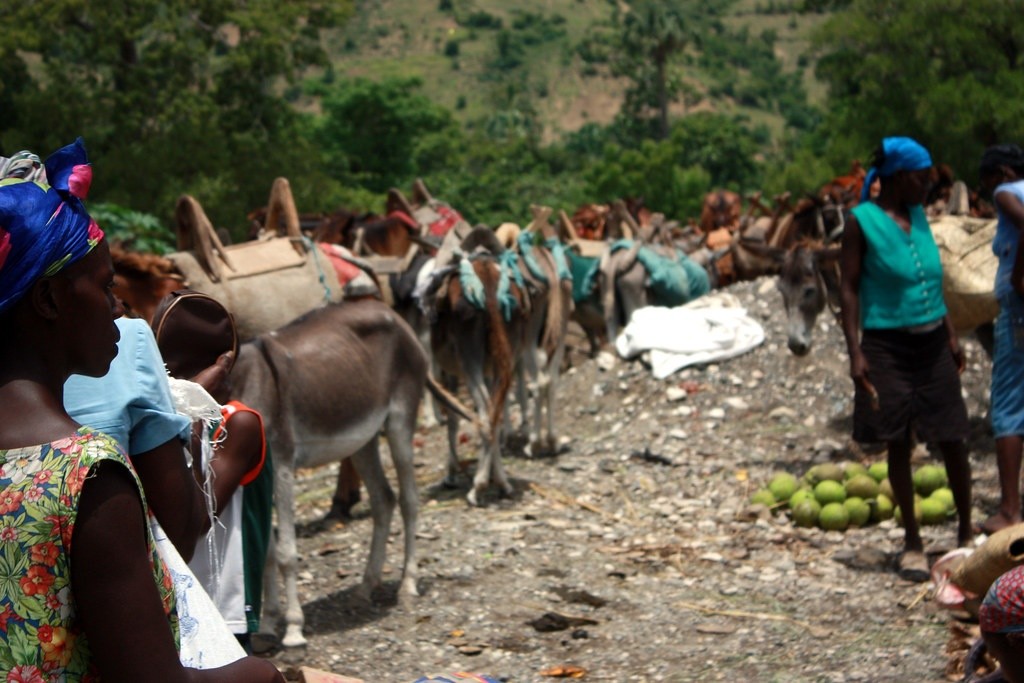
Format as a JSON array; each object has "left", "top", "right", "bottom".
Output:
[
  {"left": 972, "top": 143, "right": 1024, "bottom": 535},
  {"left": 1, "top": 143, "right": 282, "bottom": 682},
  {"left": 839, "top": 136, "right": 973, "bottom": 580},
  {"left": 934, "top": 166, "right": 969, "bottom": 216},
  {"left": 978, "top": 565, "right": 1024, "bottom": 683}
]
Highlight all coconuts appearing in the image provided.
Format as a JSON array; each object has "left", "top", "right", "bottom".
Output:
[{"left": 754, "top": 460, "right": 957, "bottom": 531}]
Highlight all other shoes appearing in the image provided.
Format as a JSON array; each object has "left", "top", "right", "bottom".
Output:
[{"left": 900, "top": 551, "right": 930, "bottom": 581}]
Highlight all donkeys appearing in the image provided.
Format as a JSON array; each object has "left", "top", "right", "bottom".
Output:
[{"left": 0, "top": 183, "right": 1024, "bottom": 667}]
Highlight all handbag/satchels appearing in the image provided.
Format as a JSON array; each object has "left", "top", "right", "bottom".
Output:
[{"left": 949, "top": 523, "right": 1024, "bottom": 592}]
